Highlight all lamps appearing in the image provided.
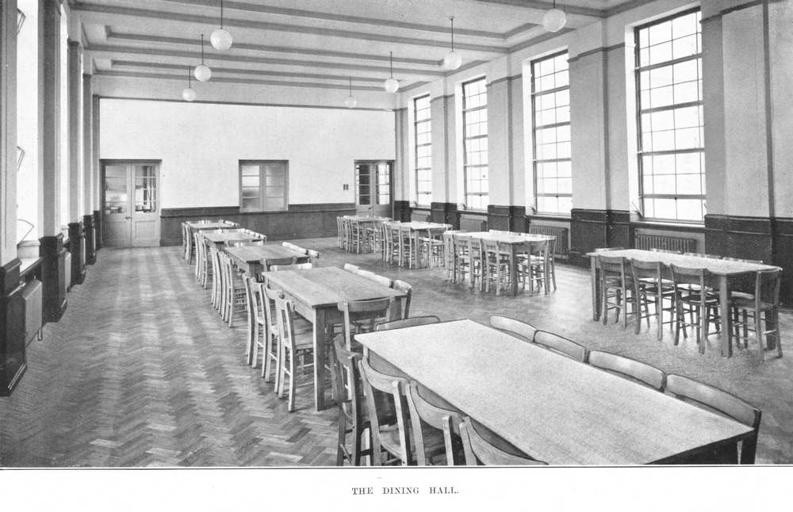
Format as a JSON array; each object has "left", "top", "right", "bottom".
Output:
[
  {"left": 179, "top": 66, "right": 198, "bottom": 102},
  {"left": 342, "top": 77, "right": 356, "bottom": 110},
  {"left": 210, "top": 0, "right": 233, "bottom": 51},
  {"left": 541, "top": 0, "right": 566, "bottom": 32},
  {"left": 191, "top": 32, "right": 213, "bottom": 83},
  {"left": 384, "top": 50, "right": 399, "bottom": 94},
  {"left": 445, "top": 16, "right": 461, "bottom": 71}
]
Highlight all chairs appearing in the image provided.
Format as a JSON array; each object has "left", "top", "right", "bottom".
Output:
[{"left": 180, "top": 214, "right": 793, "bottom": 466}]
